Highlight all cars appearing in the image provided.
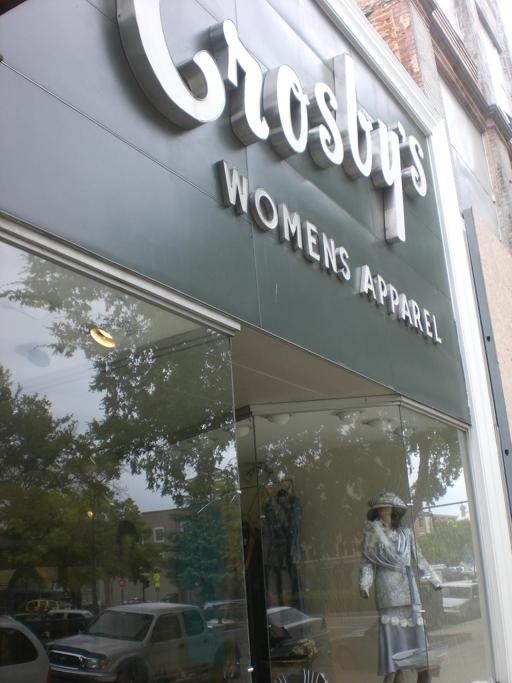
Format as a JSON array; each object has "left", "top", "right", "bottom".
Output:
[{"left": 419, "top": 563, "right": 481, "bottom": 629}]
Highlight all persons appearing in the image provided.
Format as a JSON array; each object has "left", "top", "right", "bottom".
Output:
[
  {"left": 264, "top": 488, "right": 305, "bottom": 613},
  {"left": 358, "top": 490, "right": 443, "bottom": 683}
]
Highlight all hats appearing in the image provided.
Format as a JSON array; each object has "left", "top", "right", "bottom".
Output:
[{"left": 367, "top": 492, "right": 408, "bottom": 521}]
